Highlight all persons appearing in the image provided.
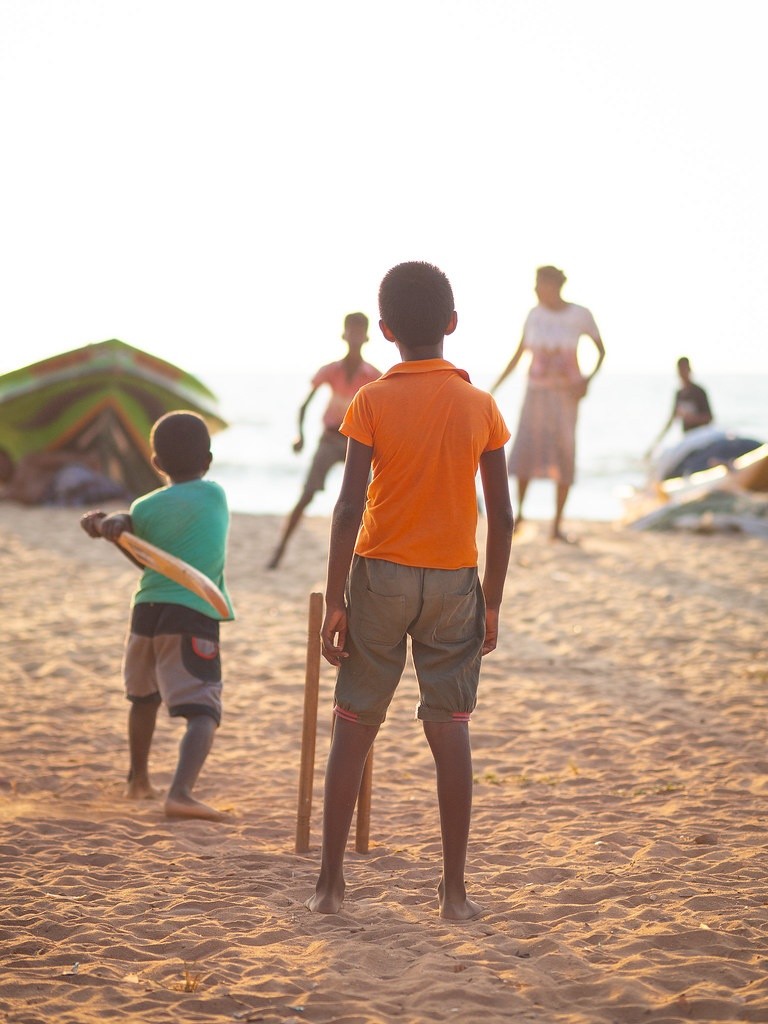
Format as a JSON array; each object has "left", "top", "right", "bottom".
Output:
[
  {"left": 1, "top": 424, "right": 113, "bottom": 503},
  {"left": 81, "top": 410, "right": 239, "bottom": 827},
  {"left": 489, "top": 265, "right": 604, "bottom": 542},
  {"left": 267, "top": 312, "right": 384, "bottom": 571},
  {"left": 651, "top": 358, "right": 713, "bottom": 532},
  {"left": 302, "top": 260, "right": 515, "bottom": 922}
]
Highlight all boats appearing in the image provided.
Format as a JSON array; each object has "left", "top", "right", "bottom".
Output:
[
  {"left": 0, "top": 339, "right": 228, "bottom": 498},
  {"left": 622, "top": 432, "right": 768, "bottom": 530}
]
